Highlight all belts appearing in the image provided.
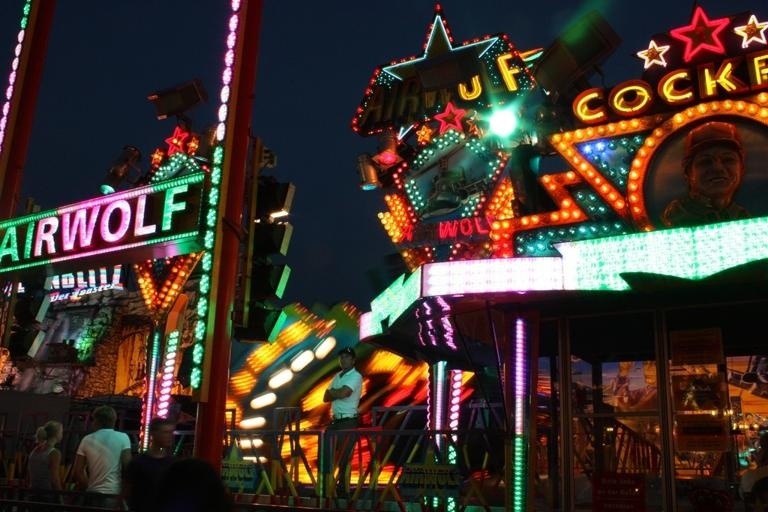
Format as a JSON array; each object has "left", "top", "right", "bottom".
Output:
[{"left": 334, "top": 413, "right": 356, "bottom": 421}]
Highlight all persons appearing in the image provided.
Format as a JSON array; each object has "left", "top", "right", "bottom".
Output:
[
  {"left": 313, "top": 347, "right": 363, "bottom": 498},
  {"left": 738, "top": 432, "right": 768, "bottom": 500},
  {"left": 658, "top": 122, "right": 753, "bottom": 228},
  {"left": 27, "top": 419, "right": 64, "bottom": 504},
  {"left": 71, "top": 404, "right": 133, "bottom": 511},
  {"left": 122, "top": 416, "right": 178, "bottom": 510}
]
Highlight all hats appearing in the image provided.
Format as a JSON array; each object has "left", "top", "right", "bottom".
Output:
[{"left": 336, "top": 347, "right": 356, "bottom": 358}]
